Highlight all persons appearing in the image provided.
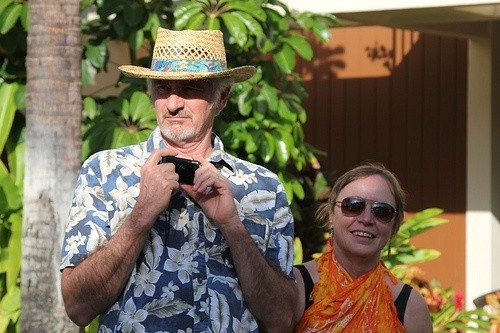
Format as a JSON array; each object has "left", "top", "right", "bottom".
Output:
[
  {"left": 58, "top": 25, "right": 299, "bottom": 333},
  {"left": 285, "top": 162, "right": 439, "bottom": 333}
]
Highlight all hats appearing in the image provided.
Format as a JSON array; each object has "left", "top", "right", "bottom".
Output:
[{"left": 118, "top": 27, "right": 257, "bottom": 83}]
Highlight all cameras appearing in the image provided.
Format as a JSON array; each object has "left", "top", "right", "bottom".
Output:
[{"left": 161, "top": 156, "right": 200, "bottom": 185}]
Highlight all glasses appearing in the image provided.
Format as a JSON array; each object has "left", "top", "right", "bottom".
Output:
[{"left": 333, "top": 197, "right": 399, "bottom": 224}]
